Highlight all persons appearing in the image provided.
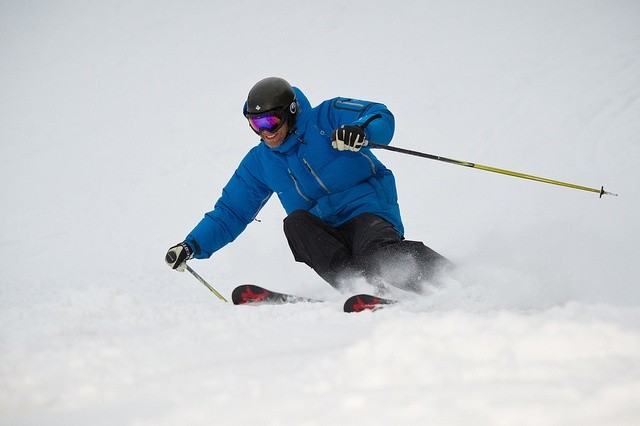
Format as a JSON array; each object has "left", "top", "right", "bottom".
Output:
[{"left": 166, "top": 76, "right": 408, "bottom": 298}]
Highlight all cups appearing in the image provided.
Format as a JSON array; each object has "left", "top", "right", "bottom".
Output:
[{"left": 243, "top": 101, "right": 293, "bottom": 135}]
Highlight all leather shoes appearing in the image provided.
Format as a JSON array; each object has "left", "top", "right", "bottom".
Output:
[
  {"left": 165, "top": 243, "right": 194, "bottom": 272},
  {"left": 331, "top": 125, "right": 365, "bottom": 152}
]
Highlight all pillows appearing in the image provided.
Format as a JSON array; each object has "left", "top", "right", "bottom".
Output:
[{"left": 247, "top": 77, "right": 297, "bottom": 148}]
